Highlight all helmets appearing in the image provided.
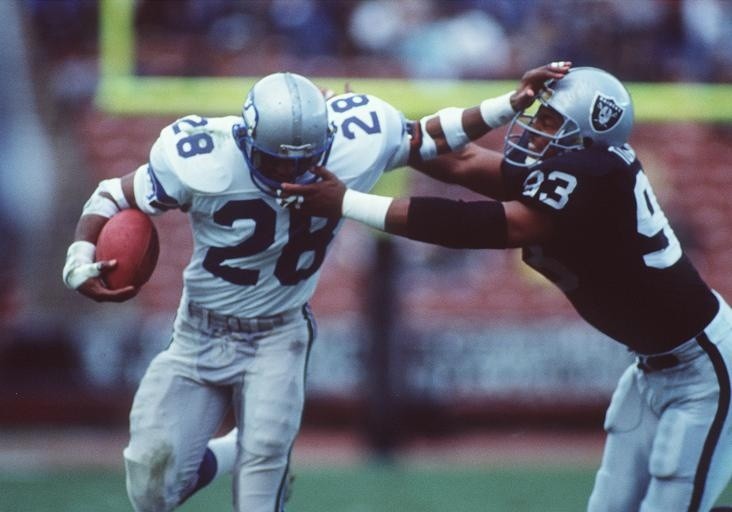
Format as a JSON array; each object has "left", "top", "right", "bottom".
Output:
[
  {"left": 504, "top": 67, "right": 634, "bottom": 168},
  {"left": 233, "top": 73, "right": 337, "bottom": 201}
]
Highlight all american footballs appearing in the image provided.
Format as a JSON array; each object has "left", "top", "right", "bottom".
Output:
[{"left": 96, "top": 209, "right": 159, "bottom": 297}]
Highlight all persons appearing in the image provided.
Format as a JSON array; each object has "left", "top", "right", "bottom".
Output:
[
  {"left": 276, "top": 66, "right": 731, "bottom": 512},
  {"left": 60, "top": 59, "right": 573, "bottom": 511}
]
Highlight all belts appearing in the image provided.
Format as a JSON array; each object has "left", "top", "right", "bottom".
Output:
[
  {"left": 636, "top": 353, "right": 681, "bottom": 371},
  {"left": 187, "top": 302, "right": 283, "bottom": 332}
]
